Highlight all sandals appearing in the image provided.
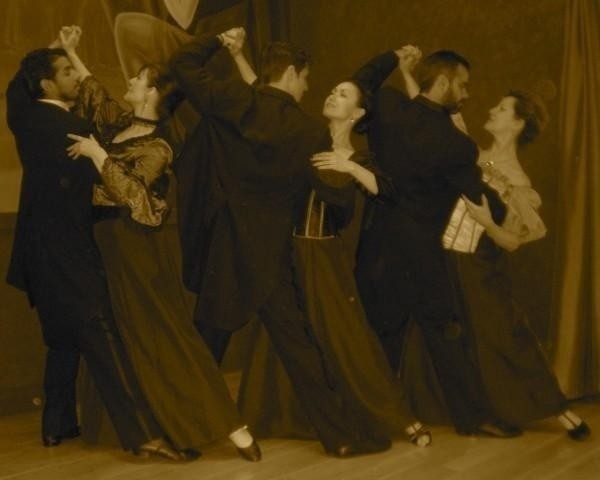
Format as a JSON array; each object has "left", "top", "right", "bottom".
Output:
[{"left": 406, "top": 423, "right": 431, "bottom": 448}]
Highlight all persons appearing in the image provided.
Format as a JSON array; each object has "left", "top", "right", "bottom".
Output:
[
  {"left": 6, "top": 24, "right": 201, "bottom": 462},
  {"left": 218, "top": 28, "right": 432, "bottom": 448},
  {"left": 59, "top": 24, "right": 261, "bottom": 462},
  {"left": 349, "top": 48, "right": 523, "bottom": 439},
  {"left": 168, "top": 28, "right": 391, "bottom": 457},
  {"left": 400, "top": 45, "right": 591, "bottom": 441}
]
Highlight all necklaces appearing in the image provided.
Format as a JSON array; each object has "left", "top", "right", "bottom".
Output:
[
  {"left": 131, "top": 117, "right": 159, "bottom": 128},
  {"left": 486, "top": 151, "right": 517, "bottom": 166}
]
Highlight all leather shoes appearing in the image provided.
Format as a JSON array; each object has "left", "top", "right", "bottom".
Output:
[
  {"left": 339, "top": 436, "right": 391, "bottom": 458},
  {"left": 43, "top": 425, "right": 81, "bottom": 448},
  {"left": 458, "top": 420, "right": 523, "bottom": 439},
  {"left": 235, "top": 439, "right": 261, "bottom": 463},
  {"left": 561, "top": 410, "right": 589, "bottom": 441},
  {"left": 134, "top": 437, "right": 203, "bottom": 462}
]
